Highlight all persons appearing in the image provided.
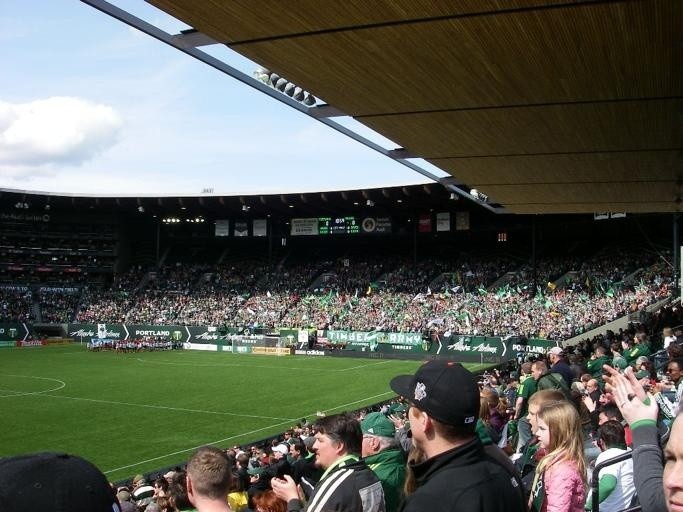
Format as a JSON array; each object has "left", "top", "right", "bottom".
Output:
[
  {"left": 1, "top": 232, "right": 309, "bottom": 333},
  {"left": 17, "top": 333, "right": 48, "bottom": 346},
  {"left": 0, "top": 418, "right": 309, "bottom": 511},
  {"left": 309, "top": 225, "right": 682, "bottom": 512},
  {"left": 86, "top": 335, "right": 182, "bottom": 355}
]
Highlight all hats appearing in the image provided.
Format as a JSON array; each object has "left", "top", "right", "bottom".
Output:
[
  {"left": 360, "top": 411, "right": 396, "bottom": 438},
  {"left": 118, "top": 470, "right": 178, "bottom": 501},
  {"left": 389, "top": 360, "right": 481, "bottom": 427},
  {"left": 271, "top": 444, "right": 289, "bottom": 455},
  {"left": 547, "top": 347, "right": 564, "bottom": 356},
  {"left": 0, "top": 452, "right": 114, "bottom": 512}
]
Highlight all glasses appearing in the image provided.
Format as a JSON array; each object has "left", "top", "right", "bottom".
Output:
[{"left": 666, "top": 369, "right": 679, "bottom": 373}]
